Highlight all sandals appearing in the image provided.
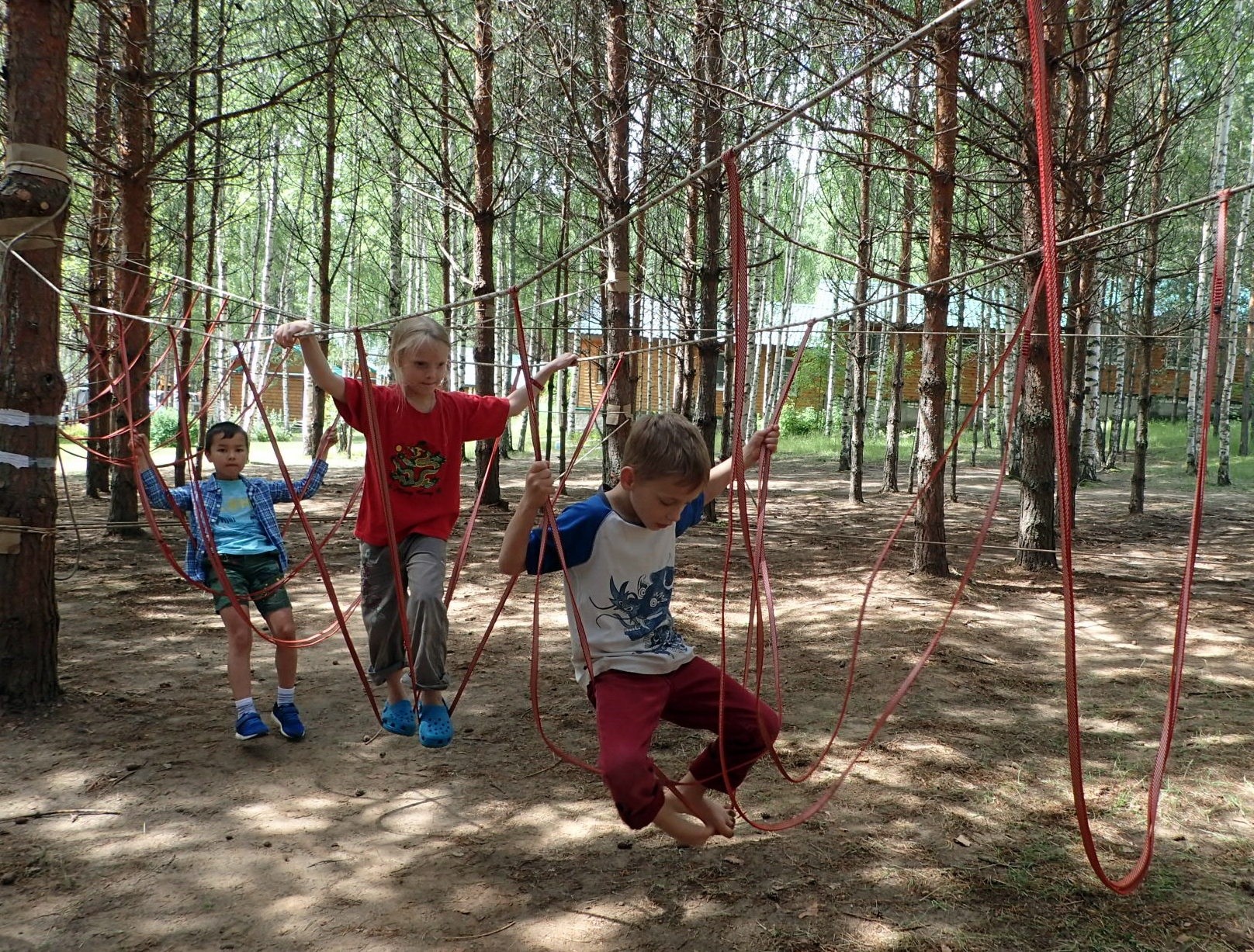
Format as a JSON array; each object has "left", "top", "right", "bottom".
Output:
[
  {"left": 417, "top": 697, "right": 453, "bottom": 748},
  {"left": 382, "top": 699, "right": 416, "bottom": 737}
]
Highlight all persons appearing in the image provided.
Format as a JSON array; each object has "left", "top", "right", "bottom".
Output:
[
  {"left": 499, "top": 413, "right": 781, "bottom": 847},
  {"left": 127, "top": 421, "right": 339, "bottom": 741},
  {"left": 273, "top": 317, "right": 579, "bottom": 747}
]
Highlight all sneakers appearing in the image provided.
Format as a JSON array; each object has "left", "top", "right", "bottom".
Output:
[
  {"left": 271, "top": 701, "right": 306, "bottom": 740},
  {"left": 235, "top": 713, "right": 270, "bottom": 741}
]
